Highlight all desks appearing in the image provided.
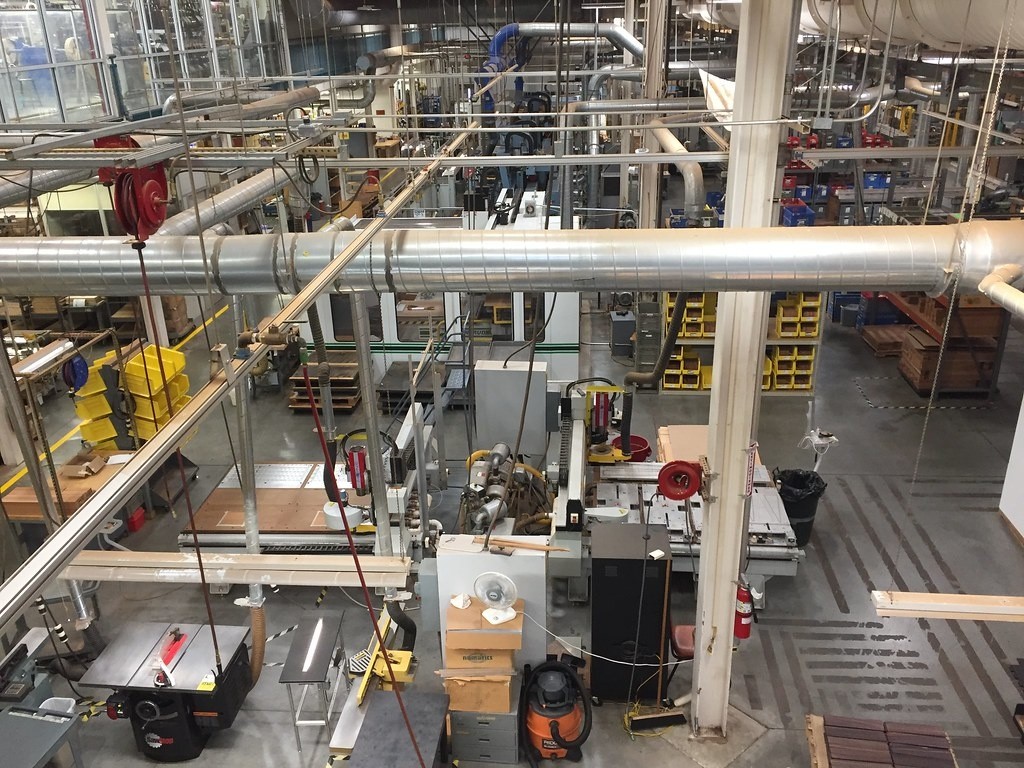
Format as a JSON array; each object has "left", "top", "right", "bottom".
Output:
[
  {"left": 279, "top": 609, "right": 350, "bottom": 752},
  {"left": 374, "top": 140, "right": 401, "bottom": 158}
]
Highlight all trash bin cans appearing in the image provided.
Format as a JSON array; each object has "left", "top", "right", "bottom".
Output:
[{"left": 772, "top": 466, "right": 828, "bottom": 545}]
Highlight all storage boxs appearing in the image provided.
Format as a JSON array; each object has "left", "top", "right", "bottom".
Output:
[
  {"left": 444, "top": 594, "right": 524, "bottom": 713},
  {"left": 669, "top": 129, "right": 949, "bottom": 227},
  {"left": 827, "top": 291, "right": 912, "bottom": 333},
  {"left": 33, "top": 697, "right": 76, "bottom": 721},
  {"left": 128, "top": 507, "right": 145, "bottom": 532},
  {"left": 61, "top": 453, "right": 106, "bottom": 479}
]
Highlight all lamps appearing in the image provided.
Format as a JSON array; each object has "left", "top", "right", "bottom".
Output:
[{"left": 869, "top": 0, "right": 1024, "bottom": 622}]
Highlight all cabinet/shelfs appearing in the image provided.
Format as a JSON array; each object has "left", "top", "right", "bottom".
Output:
[
  {"left": 451, "top": 673, "right": 523, "bottom": 764},
  {"left": 70, "top": 337, "right": 200, "bottom": 512},
  {"left": 658, "top": 292, "right": 828, "bottom": 397}
]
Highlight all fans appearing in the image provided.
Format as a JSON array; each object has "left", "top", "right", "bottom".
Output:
[{"left": 473, "top": 572, "right": 518, "bottom": 625}]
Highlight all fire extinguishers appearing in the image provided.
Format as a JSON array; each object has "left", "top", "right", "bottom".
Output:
[{"left": 729, "top": 572, "right": 759, "bottom": 639}]
